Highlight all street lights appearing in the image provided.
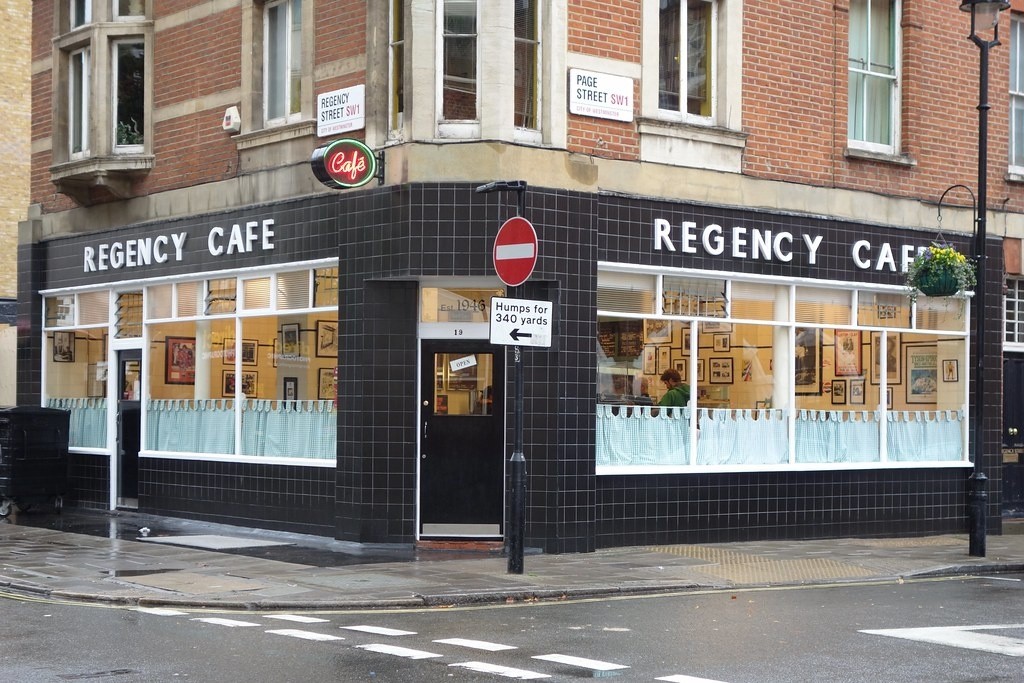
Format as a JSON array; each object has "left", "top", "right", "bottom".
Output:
[{"left": 959, "top": 0, "right": 1012, "bottom": 557}]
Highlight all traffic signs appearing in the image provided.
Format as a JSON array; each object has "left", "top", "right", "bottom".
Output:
[{"left": 488, "top": 296, "right": 553, "bottom": 347}]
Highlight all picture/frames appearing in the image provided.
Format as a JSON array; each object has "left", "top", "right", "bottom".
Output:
[
  {"left": 316, "top": 320, "right": 338, "bottom": 358},
  {"left": 164, "top": 336, "right": 196, "bottom": 385},
  {"left": 831, "top": 380, "right": 846, "bottom": 404},
  {"left": 643, "top": 319, "right": 734, "bottom": 384},
  {"left": 905, "top": 345, "right": 936, "bottom": 403},
  {"left": 283, "top": 377, "right": 297, "bottom": 411},
  {"left": 273, "top": 338, "right": 277, "bottom": 367},
  {"left": 755, "top": 401, "right": 770, "bottom": 420},
  {"left": 878, "top": 305, "right": 897, "bottom": 319},
  {"left": 850, "top": 379, "right": 865, "bottom": 404},
  {"left": 281, "top": 323, "right": 300, "bottom": 358},
  {"left": 317, "top": 368, "right": 334, "bottom": 400},
  {"left": 795, "top": 328, "right": 823, "bottom": 396},
  {"left": 437, "top": 353, "right": 477, "bottom": 392},
  {"left": 870, "top": 331, "right": 902, "bottom": 385},
  {"left": 222, "top": 370, "right": 258, "bottom": 398},
  {"left": 223, "top": 337, "right": 258, "bottom": 366},
  {"left": 878, "top": 387, "right": 893, "bottom": 410},
  {"left": 834, "top": 329, "right": 862, "bottom": 376},
  {"left": 942, "top": 359, "right": 958, "bottom": 382},
  {"left": 52, "top": 331, "right": 76, "bottom": 363}
]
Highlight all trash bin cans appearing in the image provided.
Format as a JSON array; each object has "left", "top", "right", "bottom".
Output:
[{"left": 0, "top": 404, "right": 72, "bottom": 518}]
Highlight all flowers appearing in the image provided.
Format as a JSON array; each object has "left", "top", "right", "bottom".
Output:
[{"left": 905, "top": 241, "right": 977, "bottom": 320}]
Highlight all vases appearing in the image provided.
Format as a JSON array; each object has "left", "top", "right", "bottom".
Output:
[{"left": 918, "top": 268, "right": 958, "bottom": 296}]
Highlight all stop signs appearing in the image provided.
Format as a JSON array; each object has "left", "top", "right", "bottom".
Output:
[{"left": 493, "top": 217, "right": 538, "bottom": 287}]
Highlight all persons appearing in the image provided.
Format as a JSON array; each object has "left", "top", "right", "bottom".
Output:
[
  {"left": 651, "top": 368, "right": 689, "bottom": 417},
  {"left": 473, "top": 386, "right": 492, "bottom": 414},
  {"left": 641, "top": 376, "right": 649, "bottom": 398}
]
